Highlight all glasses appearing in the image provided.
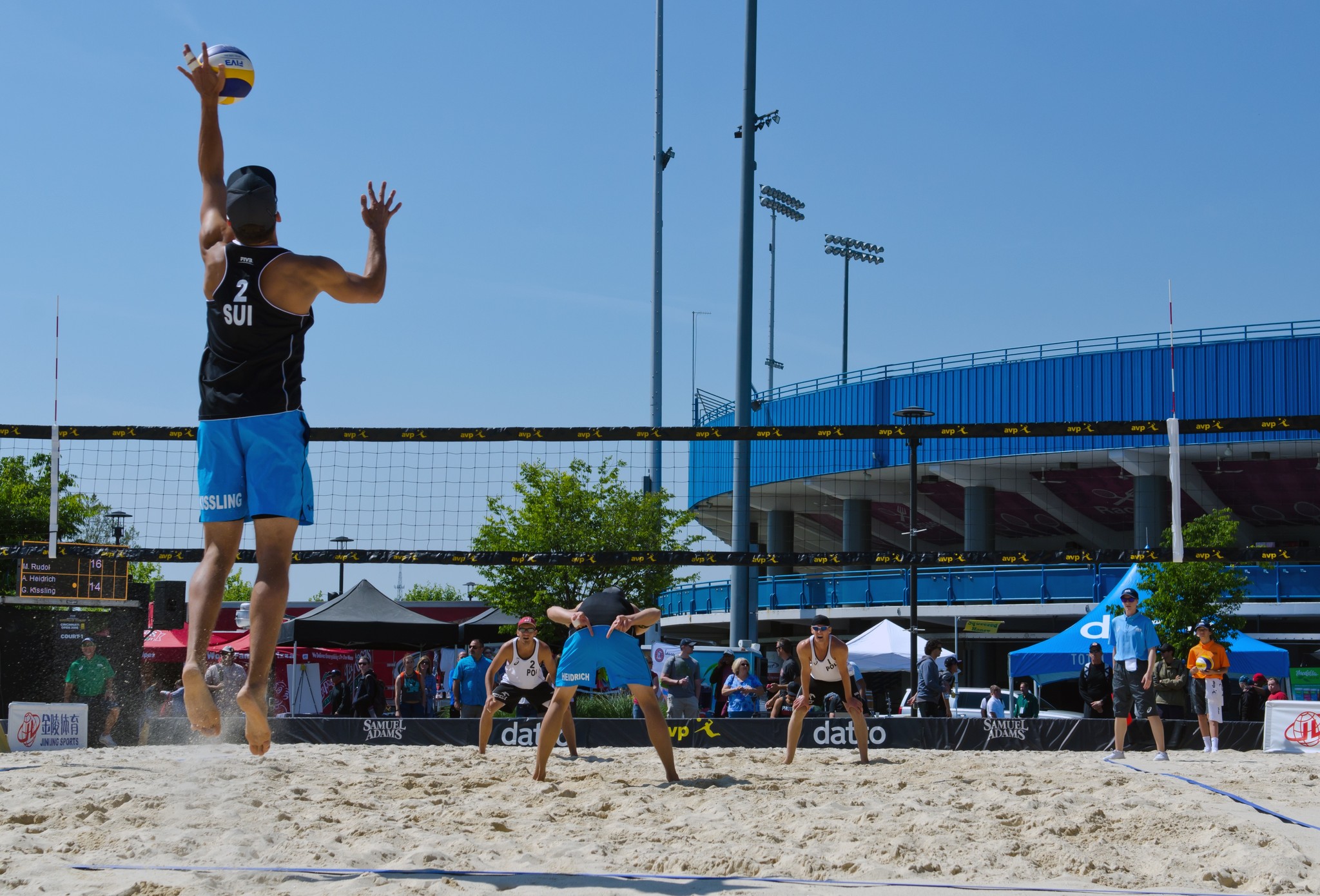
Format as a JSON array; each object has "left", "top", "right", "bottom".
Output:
[
  {"left": 811, "top": 625, "right": 830, "bottom": 630},
  {"left": 1121, "top": 597, "right": 1135, "bottom": 602},
  {"left": 741, "top": 663, "right": 749, "bottom": 666},
  {"left": 775, "top": 646, "right": 779, "bottom": 649},
  {"left": 358, "top": 662, "right": 367, "bottom": 665},
  {"left": 517, "top": 627, "right": 535, "bottom": 632},
  {"left": 81, "top": 643, "right": 95, "bottom": 647},
  {"left": 1090, "top": 649, "right": 1099, "bottom": 653},
  {"left": 420, "top": 661, "right": 429, "bottom": 665},
  {"left": 469, "top": 645, "right": 480, "bottom": 649}
]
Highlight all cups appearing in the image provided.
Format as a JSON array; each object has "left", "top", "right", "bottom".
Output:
[{"left": 875, "top": 712, "right": 879, "bottom": 717}]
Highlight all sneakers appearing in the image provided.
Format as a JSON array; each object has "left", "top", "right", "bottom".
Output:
[
  {"left": 1153, "top": 751, "right": 1169, "bottom": 761},
  {"left": 1203, "top": 747, "right": 1218, "bottom": 754},
  {"left": 99, "top": 733, "right": 116, "bottom": 748},
  {"left": 1103, "top": 748, "right": 1125, "bottom": 759}
]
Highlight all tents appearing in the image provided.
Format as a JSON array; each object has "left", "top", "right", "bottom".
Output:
[
  {"left": 143, "top": 578, "right": 569, "bottom": 719},
  {"left": 846, "top": 619, "right": 958, "bottom": 718},
  {"left": 1007, "top": 546, "right": 1292, "bottom": 719}
]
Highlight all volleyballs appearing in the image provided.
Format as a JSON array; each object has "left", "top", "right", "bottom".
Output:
[
  {"left": 1195, "top": 655, "right": 1214, "bottom": 672},
  {"left": 196, "top": 44, "right": 255, "bottom": 106}
]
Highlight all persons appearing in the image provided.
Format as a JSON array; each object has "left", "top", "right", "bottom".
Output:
[
  {"left": 1103, "top": 589, "right": 1169, "bottom": 760},
  {"left": 533, "top": 587, "right": 679, "bottom": 783},
  {"left": 64, "top": 638, "right": 122, "bottom": 748},
  {"left": 176, "top": 42, "right": 401, "bottom": 757},
  {"left": 783, "top": 615, "right": 871, "bottom": 765},
  {"left": 142, "top": 616, "right": 1288, "bottom": 756}
]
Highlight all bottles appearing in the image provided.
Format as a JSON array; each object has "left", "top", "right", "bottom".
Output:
[
  {"left": 941, "top": 681, "right": 950, "bottom": 699},
  {"left": 682, "top": 676, "right": 689, "bottom": 689}
]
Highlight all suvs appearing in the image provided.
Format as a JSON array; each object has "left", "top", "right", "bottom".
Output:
[{"left": 898, "top": 686, "right": 1084, "bottom": 719}]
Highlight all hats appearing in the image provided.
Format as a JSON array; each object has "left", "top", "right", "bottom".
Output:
[
  {"left": 787, "top": 682, "right": 800, "bottom": 696},
  {"left": 225, "top": 165, "right": 278, "bottom": 245},
  {"left": 328, "top": 669, "right": 341, "bottom": 675},
  {"left": 1253, "top": 673, "right": 1266, "bottom": 681},
  {"left": 219, "top": 646, "right": 234, "bottom": 652},
  {"left": 724, "top": 649, "right": 735, "bottom": 656},
  {"left": 812, "top": 615, "right": 830, "bottom": 625},
  {"left": 81, "top": 637, "right": 94, "bottom": 644},
  {"left": 1195, "top": 622, "right": 1209, "bottom": 629},
  {"left": 945, "top": 656, "right": 962, "bottom": 667},
  {"left": 1120, "top": 588, "right": 1138, "bottom": 599},
  {"left": 518, "top": 617, "right": 536, "bottom": 628},
  {"left": 680, "top": 639, "right": 697, "bottom": 646},
  {"left": 1156, "top": 643, "right": 1175, "bottom": 654},
  {"left": 1089, "top": 642, "right": 1101, "bottom": 650}
]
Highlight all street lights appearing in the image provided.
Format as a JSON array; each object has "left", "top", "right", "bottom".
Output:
[
  {"left": 329, "top": 536, "right": 354, "bottom": 596},
  {"left": 758, "top": 184, "right": 806, "bottom": 401},
  {"left": 824, "top": 233, "right": 885, "bottom": 388},
  {"left": 104, "top": 510, "right": 133, "bottom": 546},
  {"left": 892, "top": 405, "right": 936, "bottom": 718}
]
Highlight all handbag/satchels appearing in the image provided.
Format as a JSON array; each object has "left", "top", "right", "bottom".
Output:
[{"left": 721, "top": 700, "right": 729, "bottom": 717}]
